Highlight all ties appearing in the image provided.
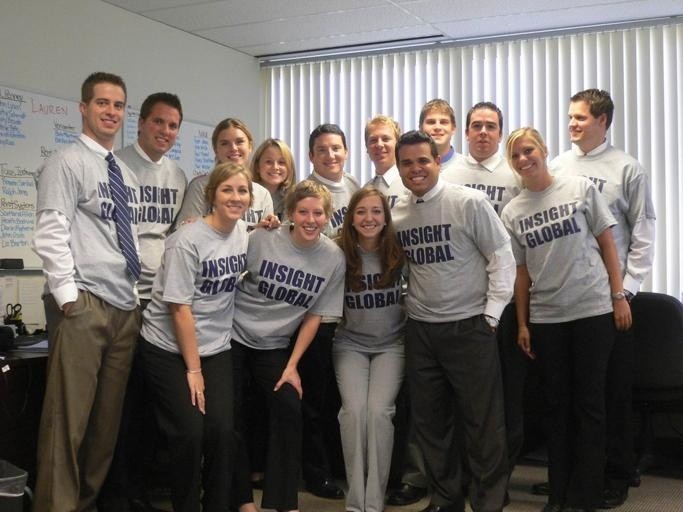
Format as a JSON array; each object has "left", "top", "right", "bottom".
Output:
[{"left": 103, "top": 152, "right": 141, "bottom": 281}]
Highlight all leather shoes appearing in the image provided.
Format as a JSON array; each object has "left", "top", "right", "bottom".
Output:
[
  {"left": 597, "top": 482, "right": 628, "bottom": 507},
  {"left": 303, "top": 471, "right": 344, "bottom": 498},
  {"left": 530, "top": 481, "right": 550, "bottom": 493},
  {"left": 630, "top": 468, "right": 640, "bottom": 487},
  {"left": 385, "top": 482, "right": 428, "bottom": 505},
  {"left": 417, "top": 498, "right": 467, "bottom": 512}
]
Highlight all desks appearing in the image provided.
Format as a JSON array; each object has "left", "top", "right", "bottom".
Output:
[{"left": 0, "top": 339, "right": 48, "bottom": 510}]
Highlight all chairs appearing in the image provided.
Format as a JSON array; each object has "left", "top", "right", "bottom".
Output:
[
  {"left": 495, "top": 299, "right": 532, "bottom": 455},
  {"left": 627, "top": 291, "right": 683, "bottom": 475}
]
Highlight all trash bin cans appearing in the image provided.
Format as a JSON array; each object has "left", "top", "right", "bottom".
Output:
[{"left": 0, "top": 460, "right": 33, "bottom": 512}]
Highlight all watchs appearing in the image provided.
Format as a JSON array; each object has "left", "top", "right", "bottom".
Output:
[
  {"left": 610, "top": 290, "right": 626, "bottom": 300},
  {"left": 483, "top": 314, "right": 498, "bottom": 329}
]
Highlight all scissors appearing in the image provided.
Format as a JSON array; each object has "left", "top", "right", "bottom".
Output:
[{"left": 6, "top": 303, "right": 21, "bottom": 318}]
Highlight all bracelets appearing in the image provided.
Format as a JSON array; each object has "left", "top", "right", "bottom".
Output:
[{"left": 184, "top": 368, "right": 202, "bottom": 374}]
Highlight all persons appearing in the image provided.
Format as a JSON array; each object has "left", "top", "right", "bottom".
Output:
[
  {"left": 173, "top": 118, "right": 275, "bottom": 233},
  {"left": 250, "top": 140, "right": 297, "bottom": 220},
  {"left": 95, "top": 92, "right": 193, "bottom": 511},
  {"left": 301, "top": 124, "right": 362, "bottom": 497},
  {"left": 140, "top": 162, "right": 281, "bottom": 511},
  {"left": 450, "top": 101, "right": 530, "bottom": 506},
  {"left": 28, "top": 72, "right": 144, "bottom": 511},
  {"left": 419, "top": 98, "right": 468, "bottom": 188},
  {"left": 327, "top": 185, "right": 407, "bottom": 512},
  {"left": 527, "top": 90, "right": 656, "bottom": 507},
  {"left": 362, "top": 117, "right": 431, "bottom": 506},
  {"left": 181, "top": 179, "right": 348, "bottom": 512},
  {"left": 385, "top": 131, "right": 517, "bottom": 511},
  {"left": 494, "top": 126, "right": 633, "bottom": 512}
]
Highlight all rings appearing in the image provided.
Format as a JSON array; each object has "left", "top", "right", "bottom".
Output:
[{"left": 196, "top": 391, "right": 201, "bottom": 395}]
